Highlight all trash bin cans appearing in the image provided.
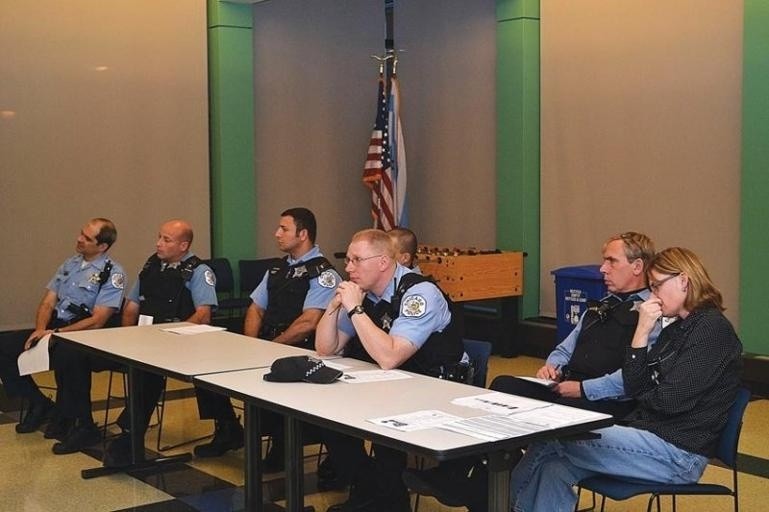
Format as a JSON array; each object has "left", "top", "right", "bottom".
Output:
[{"left": 551, "top": 266, "right": 608, "bottom": 346}]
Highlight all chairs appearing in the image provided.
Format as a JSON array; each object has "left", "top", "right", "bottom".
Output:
[
  {"left": 196, "top": 251, "right": 351, "bottom": 336},
  {"left": 18, "top": 295, "right": 505, "bottom": 512},
  {"left": 573, "top": 259, "right": 751, "bottom": 512}
]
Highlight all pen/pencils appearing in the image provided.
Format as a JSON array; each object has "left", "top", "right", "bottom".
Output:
[
  {"left": 549, "top": 364, "right": 560, "bottom": 380},
  {"left": 329, "top": 303, "right": 341, "bottom": 315}
]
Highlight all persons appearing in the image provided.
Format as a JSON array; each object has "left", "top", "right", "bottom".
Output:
[
  {"left": 410, "top": 232, "right": 664, "bottom": 507},
  {"left": 52, "top": 220, "right": 220, "bottom": 474},
  {"left": 509, "top": 246, "right": 743, "bottom": 511},
  {"left": 315, "top": 229, "right": 418, "bottom": 490},
  {"left": 193, "top": 207, "right": 344, "bottom": 473},
  {"left": 315, "top": 230, "right": 475, "bottom": 512},
  {"left": 16, "top": 218, "right": 127, "bottom": 439}
]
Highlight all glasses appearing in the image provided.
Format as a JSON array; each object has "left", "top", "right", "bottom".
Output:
[
  {"left": 345, "top": 255, "right": 384, "bottom": 264},
  {"left": 652, "top": 274, "right": 680, "bottom": 289}
]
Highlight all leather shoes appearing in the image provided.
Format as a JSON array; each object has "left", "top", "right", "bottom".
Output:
[
  {"left": 194, "top": 417, "right": 244, "bottom": 457},
  {"left": 103, "top": 429, "right": 132, "bottom": 460},
  {"left": 16, "top": 397, "right": 101, "bottom": 454},
  {"left": 262, "top": 436, "right": 284, "bottom": 472},
  {"left": 319, "top": 453, "right": 467, "bottom": 511}
]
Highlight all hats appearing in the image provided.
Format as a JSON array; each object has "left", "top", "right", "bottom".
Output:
[{"left": 264, "top": 357, "right": 342, "bottom": 384}]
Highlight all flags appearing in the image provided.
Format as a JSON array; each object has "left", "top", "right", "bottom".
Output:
[{"left": 360, "top": 71, "right": 408, "bottom": 231}]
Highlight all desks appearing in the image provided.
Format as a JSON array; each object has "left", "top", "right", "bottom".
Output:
[{"left": 49, "top": 322, "right": 611, "bottom": 512}]
